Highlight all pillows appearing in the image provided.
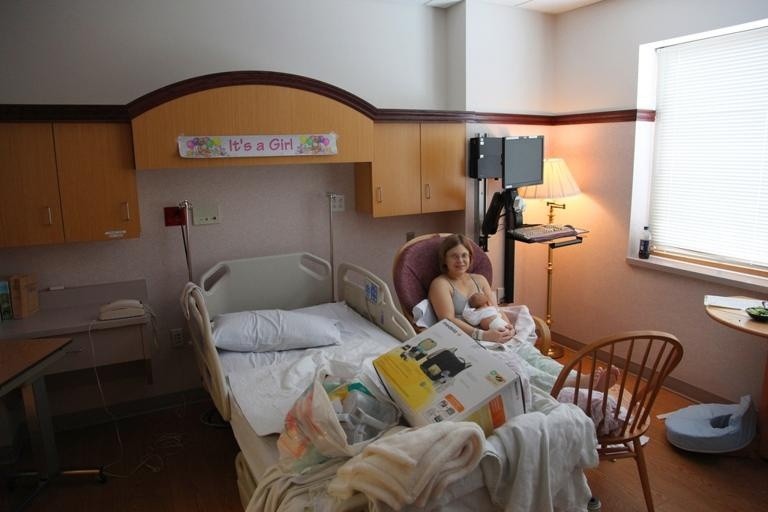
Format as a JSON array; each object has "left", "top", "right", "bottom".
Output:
[{"left": 209, "top": 309, "right": 341, "bottom": 351}]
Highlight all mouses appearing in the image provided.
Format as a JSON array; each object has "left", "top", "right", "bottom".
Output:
[{"left": 562, "top": 224, "right": 575, "bottom": 232}]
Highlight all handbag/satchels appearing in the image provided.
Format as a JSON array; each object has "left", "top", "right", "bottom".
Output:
[{"left": 278, "top": 361, "right": 402, "bottom": 475}]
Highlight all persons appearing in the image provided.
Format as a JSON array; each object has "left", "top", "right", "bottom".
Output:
[
  {"left": 462, "top": 292, "right": 514, "bottom": 334},
  {"left": 426, "top": 233, "right": 621, "bottom": 393}
]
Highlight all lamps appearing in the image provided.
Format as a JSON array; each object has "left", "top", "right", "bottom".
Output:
[{"left": 515, "top": 156, "right": 582, "bottom": 360}]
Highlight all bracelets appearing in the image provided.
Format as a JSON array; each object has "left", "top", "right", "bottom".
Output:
[{"left": 472, "top": 326, "right": 485, "bottom": 341}]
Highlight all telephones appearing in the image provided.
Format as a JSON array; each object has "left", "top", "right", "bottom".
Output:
[{"left": 97, "top": 299, "right": 147, "bottom": 320}]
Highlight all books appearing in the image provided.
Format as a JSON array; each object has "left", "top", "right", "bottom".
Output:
[
  {"left": 0, "top": 278, "right": 13, "bottom": 321},
  {"left": 9, "top": 272, "right": 40, "bottom": 319}
]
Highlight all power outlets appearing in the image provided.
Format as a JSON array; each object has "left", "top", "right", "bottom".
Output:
[{"left": 169, "top": 327, "right": 184, "bottom": 348}]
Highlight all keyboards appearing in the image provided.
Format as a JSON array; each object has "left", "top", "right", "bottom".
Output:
[{"left": 514, "top": 225, "right": 561, "bottom": 239}]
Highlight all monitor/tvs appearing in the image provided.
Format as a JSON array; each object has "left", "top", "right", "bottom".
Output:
[{"left": 502, "top": 135, "right": 544, "bottom": 190}]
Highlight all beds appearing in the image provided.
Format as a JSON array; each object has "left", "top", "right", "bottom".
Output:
[{"left": 184, "top": 252, "right": 578, "bottom": 510}]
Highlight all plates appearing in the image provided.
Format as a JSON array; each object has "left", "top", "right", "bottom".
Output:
[{"left": 745, "top": 307, "right": 768, "bottom": 321}]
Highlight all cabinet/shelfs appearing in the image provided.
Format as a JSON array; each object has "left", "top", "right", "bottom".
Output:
[
  {"left": 0, "top": 100, "right": 140, "bottom": 251},
  {"left": 354, "top": 108, "right": 468, "bottom": 221}
]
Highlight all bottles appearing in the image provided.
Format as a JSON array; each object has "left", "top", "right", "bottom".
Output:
[{"left": 639, "top": 227, "right": 651, "bottom": 258}]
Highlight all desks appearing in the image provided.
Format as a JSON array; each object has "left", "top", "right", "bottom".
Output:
[
  {"left": 1, "top": 280, "right": 150, "bottom": 478},
  {"left": 704, "top": 295, "right": 768, "bottom": 466}
]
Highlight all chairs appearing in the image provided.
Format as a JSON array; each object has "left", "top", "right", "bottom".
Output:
[
  {"left": 548, "top": 323, "right": 686, "bottom": 512},
  {"left": 392, "top": 233, "right": 550, "bottom": 361}
]
